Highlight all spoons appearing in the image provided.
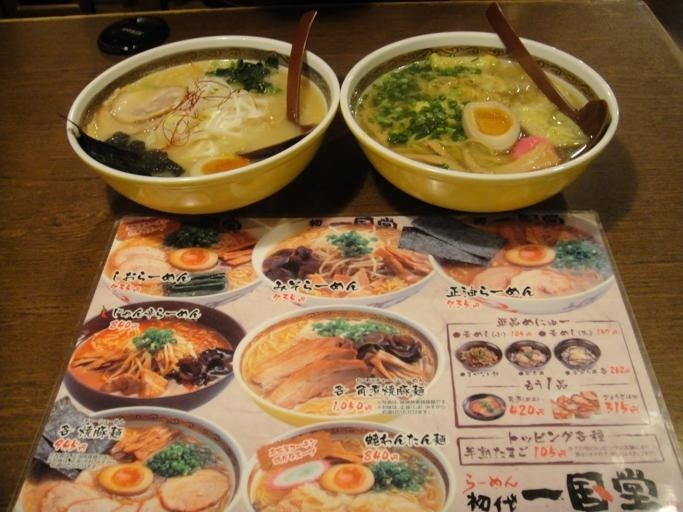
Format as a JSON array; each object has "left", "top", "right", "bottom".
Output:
[
  {"left": 484, "top": 3, "right": 611, "bottom": 164},
  {"left": 235, "top": 10, "right": 320, "bottom": 158}
]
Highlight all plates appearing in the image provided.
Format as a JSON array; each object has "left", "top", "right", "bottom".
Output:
[{"left": 94, "top": 16, "right": 171, "bottom": 54}]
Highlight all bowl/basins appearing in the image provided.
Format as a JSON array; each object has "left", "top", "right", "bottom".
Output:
[
  {"left": 38, "top": 407, "right": 246, "bottom": 512},
  {"left": 63, "top": 301, "right": 246, "bottom": 418},
  {"left": 102, "top": 223, "right": 265, "bottom": 308},
  {"left": 232, "top": 305, "right": 444, "bottom": 427},
  {"left": 421, "top": 218, "right": 618, "bottom": 316},
  {"left": 244, "top": 420, "right": 454, "bottom": 511},
  {"left": 67, "top": 34, "right": 340, "bottom": 215},
  {"left": 456, "top": 334, "right": 606, "bottom": 425},
  {"left": 251, "top": 221, "right": 435, "bottom": 313},
  {"left": 341, "top": 32, "right": 618, "bottom": 216}
]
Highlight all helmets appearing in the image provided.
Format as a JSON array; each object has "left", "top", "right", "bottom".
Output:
[{"left": 94, "top": 16, "right": 171, "bottom": 54}]
